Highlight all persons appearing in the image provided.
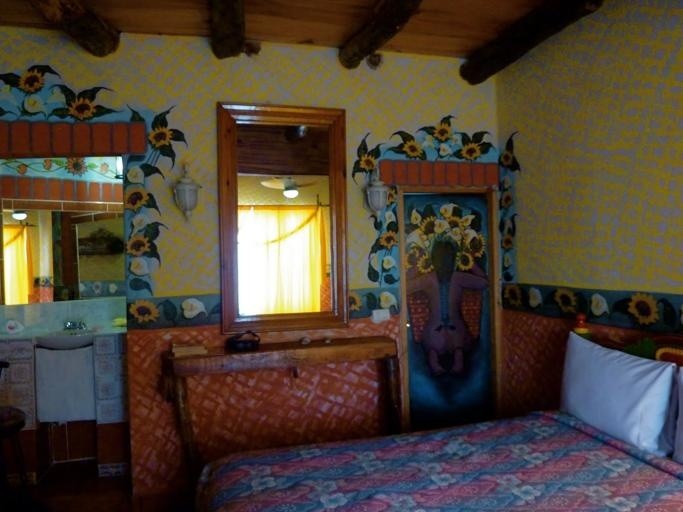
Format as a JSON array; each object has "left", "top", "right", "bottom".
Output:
[{"left": 406, "top": 241, "right": 489, "bottom": 374}]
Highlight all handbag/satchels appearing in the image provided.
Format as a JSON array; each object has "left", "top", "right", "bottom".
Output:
[{"left": 226, "top": 331, "right": 260, "bottom": 352}]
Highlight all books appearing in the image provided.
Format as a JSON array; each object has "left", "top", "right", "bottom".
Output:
[{"left": 172, "top": 343, "right": 226, "bottom": 357}]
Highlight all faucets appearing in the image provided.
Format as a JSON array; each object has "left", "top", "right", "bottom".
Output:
[{"left": 63, "top": 320, "right": 78, "bottom": 330}]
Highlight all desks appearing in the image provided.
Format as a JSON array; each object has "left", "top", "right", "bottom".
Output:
[{"left": 160, "top": 336, "right": 405, "bottom": 466}]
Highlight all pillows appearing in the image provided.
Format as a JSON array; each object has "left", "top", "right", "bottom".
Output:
[{"left": 556, "top": 327, "right": 675, "bottom": 460}]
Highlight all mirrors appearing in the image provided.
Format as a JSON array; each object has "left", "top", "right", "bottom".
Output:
[
  {"left": 0, "top": 153, "right": 127, "bottom": 340},
  {"left": 214, "top": 97, "right": 351, "bottom": 336}
]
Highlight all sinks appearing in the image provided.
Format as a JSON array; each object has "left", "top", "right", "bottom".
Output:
[{"left": 34, "top": 332, "right": 92, "bottom": 349}]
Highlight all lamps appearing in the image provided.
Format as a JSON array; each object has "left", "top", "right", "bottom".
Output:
[
  {"left": 364, "top": 167, "right": 390, "bottom": 224},
  {"left": 257, "top": 174, "right": 318, "bottom": 200},
  {"left": 173, "top": 162, "right": 202, "bottom": 221}
]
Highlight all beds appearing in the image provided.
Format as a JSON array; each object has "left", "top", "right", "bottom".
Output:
[{"left": 200, "top": 336, "right": 683, "bottom": 512}]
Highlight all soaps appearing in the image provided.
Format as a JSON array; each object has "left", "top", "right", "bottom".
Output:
[{"left": 112, "top": 317, "right": 127, "bottom": 326}]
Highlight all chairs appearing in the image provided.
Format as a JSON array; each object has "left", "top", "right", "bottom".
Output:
[{"left": 0, "top": 361, "right": 32, "bottom": 512}]
